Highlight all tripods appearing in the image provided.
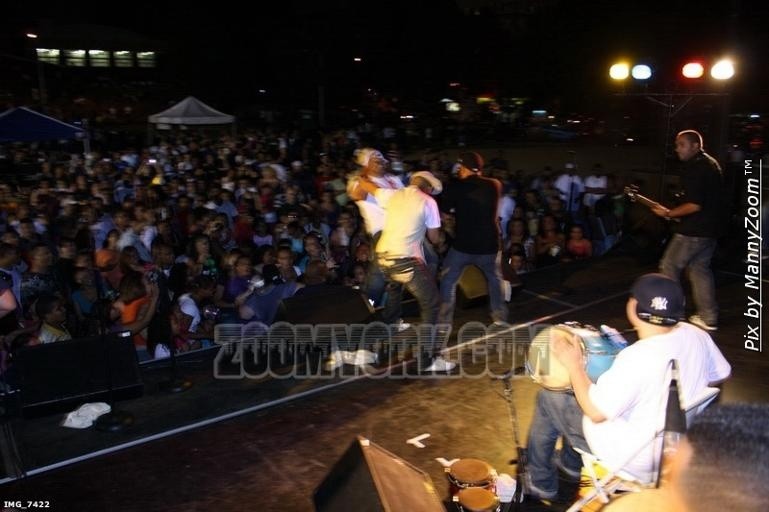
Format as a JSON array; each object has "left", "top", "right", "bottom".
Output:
[{"left": 501, "top": 387, "right": 566, "bottom": 512}]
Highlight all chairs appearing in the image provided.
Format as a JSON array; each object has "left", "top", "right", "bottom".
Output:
[{"left": 565, "top": 358, "right": 720, "bottom": 512}]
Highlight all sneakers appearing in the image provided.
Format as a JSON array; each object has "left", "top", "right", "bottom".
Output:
[
  {"left": 398, "top": 322, "right": 410, "bottom": 332},
  {"left": 520, "top": 468, "right": 557, "bottom": 499},
  {"left": 554, "top": 448, "right": 580, "bottom": 485},
  {"left": 416, "top": 356, "right": 457, "bottom": 372},
  {"left": 688, "top": 314, "right": 718, "bottom": 331}
]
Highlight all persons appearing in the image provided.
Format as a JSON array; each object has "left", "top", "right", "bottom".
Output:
[
  {"left": 651, "top": 130, "right": 728, "bottom": 331},
  {"left": 524, "top": 274, "right": 731, "bottom": 500},
  {"left": 0, "top": 87, "right": 629, "bottom": 372}
]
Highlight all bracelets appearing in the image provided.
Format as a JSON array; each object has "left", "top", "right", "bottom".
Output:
[{"left": 664, "top": 209, "right": 672, "bottom": 221}]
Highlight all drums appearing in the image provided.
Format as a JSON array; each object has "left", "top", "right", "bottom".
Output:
[
  {"left": 524, "top": 324, "right": 628, "bottom": 392},
  {"left": 455, "top": 487, "right": 498, "bottom": 512},
  {"left": 448, "top": 458, "right": 497, "bottom": 489}
]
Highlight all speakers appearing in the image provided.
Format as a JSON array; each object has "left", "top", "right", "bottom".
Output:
[
  {"left": 435, "top": 256, "right": 522, "bottom": 309},
  {"left": 275, "top": 283, "right": 375, "bottom": 350},
  {"left": 312, "top": 435, "right": 448, "bottom": 512},
  {"left": 16, "top": 329, "right": 145, "bottom": 420}
]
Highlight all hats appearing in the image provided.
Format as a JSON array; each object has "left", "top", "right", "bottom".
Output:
[
  {"left": 408, "top": 170, "right": 443, "bottom": 196},
  {"left": 456, "top": 150, "right": 485, "bottom": 172},
  {"left": 630, "top": 272, "right": 687, "bottom": 327}
]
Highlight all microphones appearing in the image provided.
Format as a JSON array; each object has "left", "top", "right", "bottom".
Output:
[{"left": 500, "top": 367, "right": 525, "bottom": 381}]
